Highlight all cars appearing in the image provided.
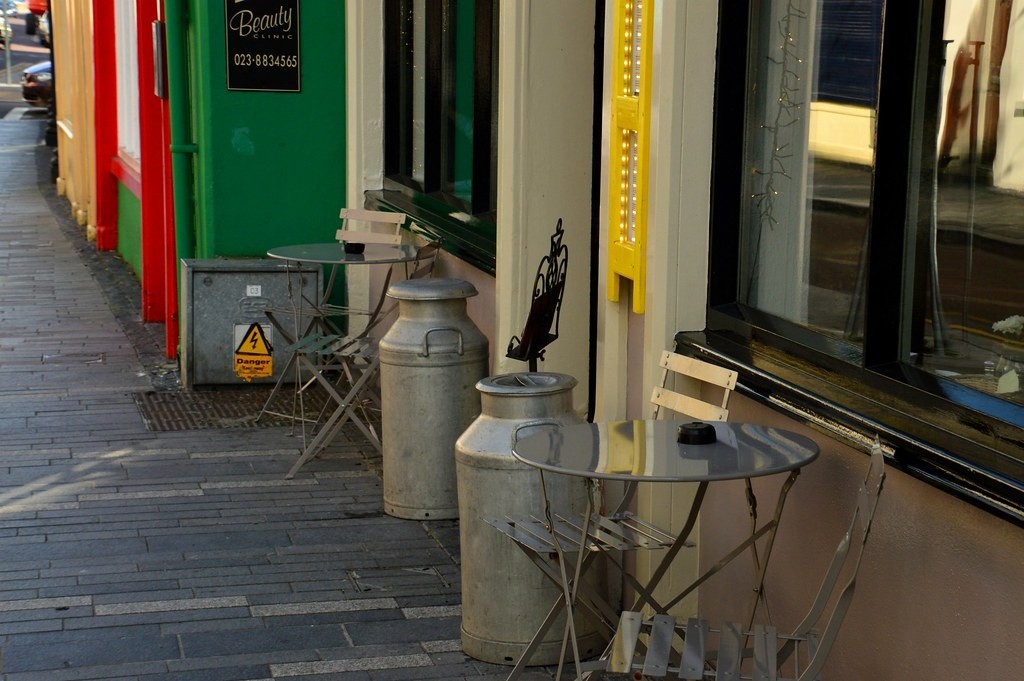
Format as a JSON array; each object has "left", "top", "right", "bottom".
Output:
[
  {"left": 26, "top": 0, "right": 52, "bottom": 47},
  {"left": 0, "top": 0, "right": 18, "bottom": 17},
  {"left": 22, "top": 62, "right": 54, "bottom": 108},
  {"left": 0, "top": 16, "right": 14, "bottom": 47}
]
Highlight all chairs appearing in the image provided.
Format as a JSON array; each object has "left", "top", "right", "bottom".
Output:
[
  {"left": 255, "top": 209, "right": 406, "bottom": 434},
  {"left": 481, "top": 351, "right": 738, "bottom": 681},
  {"left": 284, "top": 237, "right": 444, "bottom": 477},
  {"left": 605, "top": 432, "right": 887, "bottom": 681}
]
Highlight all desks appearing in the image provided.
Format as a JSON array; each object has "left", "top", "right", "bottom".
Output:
[
  {"left": 266, "top": 242, "right": 434, "bottom": 435},
  {"left": 512, "top": 421, "right": 819, "bottom": 681}
]
